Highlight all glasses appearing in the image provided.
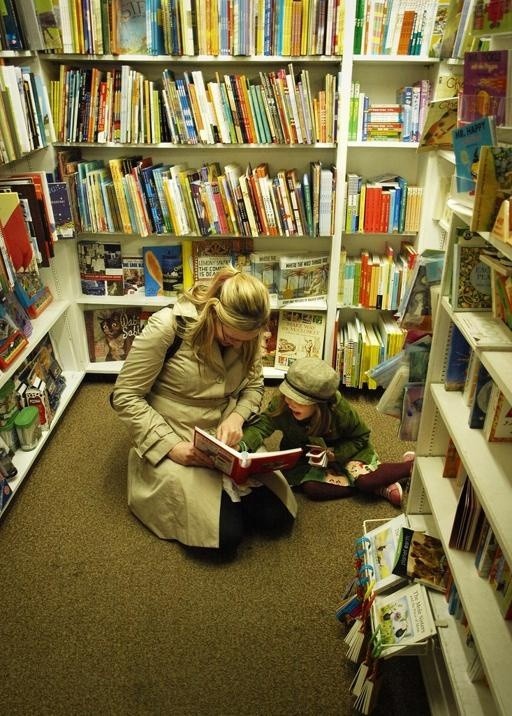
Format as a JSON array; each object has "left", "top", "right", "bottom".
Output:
[{"left": 222, "top": 325, "right": 258, "bottom": 345}]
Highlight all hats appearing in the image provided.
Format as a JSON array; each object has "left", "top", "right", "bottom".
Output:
[{"left": 279, "top": 356, "right": 340, "bottom": 405}]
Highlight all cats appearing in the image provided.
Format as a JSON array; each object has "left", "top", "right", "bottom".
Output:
[{"left": 97, "top": 311, "right": 135, "bottom": 361}]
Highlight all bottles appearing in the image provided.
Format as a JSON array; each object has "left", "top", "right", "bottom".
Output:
[{"left": 1, "top": 448, "right": 18, "bottom": 480}]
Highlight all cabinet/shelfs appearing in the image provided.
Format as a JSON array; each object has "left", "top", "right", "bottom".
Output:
[
  {"left": 1, "top": 0, "right": 512, "bottom": 715},
  {"left": 112, "top": 384, "right": 121, "bottom": 386}
]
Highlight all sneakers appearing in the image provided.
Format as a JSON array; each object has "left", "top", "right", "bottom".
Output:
[
  {"left": 374, "top": 481, "right": 403, "bottom": 504},
  {"left": 403, "top": 451, "right": 415, "bottom": 463}
]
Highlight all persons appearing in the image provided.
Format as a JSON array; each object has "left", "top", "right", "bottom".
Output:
[
  {"left": 228, "top": 355, "right": 416, "bottom": 507},
  {"left": 110, "top": 262, "right": 299, "bottom": 566}
]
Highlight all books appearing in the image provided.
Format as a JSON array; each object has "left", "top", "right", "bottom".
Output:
[
  {"left": 339, "top": 527, "right": 483, "bottom": 715},
  {"left": 349, "top": 80, "right": 429, "bottom": 141},
  {"left": 58, "top": 151, "right": 337, "bottom": 238},
  {"left": 0, "top": 0, "right": 511, "bottom": 57},
  {"left": 343, "top": 172, "right": 422, "bottom": 233},
  {"left": 445, "top": 324, "right": 511, "bottom": 442},
  {"left": 84, "top": 307, "right": 326, "bottom": 371},
  {"left": 78, "top": 238, "right": 329, "bottom": 307},
  {"left": 338, "top": 241, "right": 418, "bottom": 311},
  {"left": 1, "top": 171, "right": 75, "bottom": 372},
  {"left": 1, "top": 65, "right": 339, "bottom": 165},
  {"left": 333, "top": 309, "right": 408, "bottom": 389},
  {"left": 191, "top": 427, "right": 303, "bottom": 484},
  {"left": 418, "top": 49, "right": 512, "bottom": 350},
  {"left": 443, "top": 437, "right": 511, "bottom": 621}
]
